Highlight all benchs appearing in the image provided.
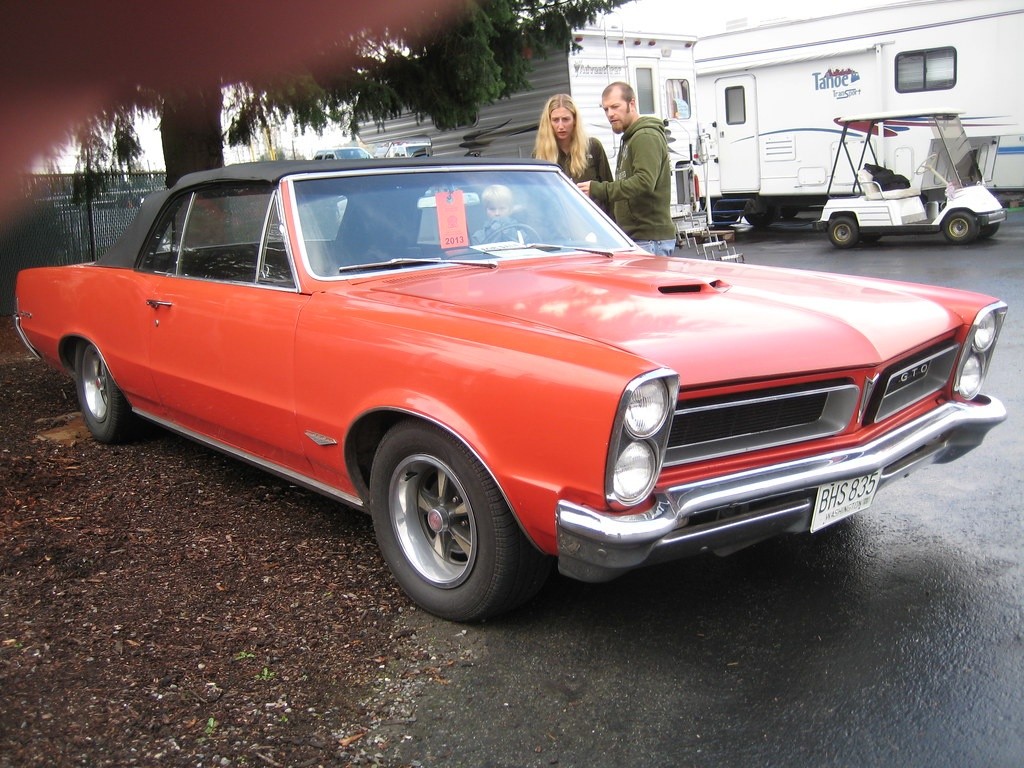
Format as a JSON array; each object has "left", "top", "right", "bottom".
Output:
[{"left": 858, "top": 166, "right": 920, "bottom": 201}]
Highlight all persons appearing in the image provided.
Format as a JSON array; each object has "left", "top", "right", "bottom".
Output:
[
  {"left": 578, "top": 82, "right": 676, "bottom": 258},
  {"left": 532, "top": 92, "right": 615, "bottom": 226},
  {"left": 472, "top": 185, "right": 533, "bottom": 245}
]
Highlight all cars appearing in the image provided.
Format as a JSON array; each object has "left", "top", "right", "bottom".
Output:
[
  {"left": 12, "top": 158, "right": 1011, "bottom": 627},
  {"left": 313, "top": 148, "right": 373, "bottom": 160}
]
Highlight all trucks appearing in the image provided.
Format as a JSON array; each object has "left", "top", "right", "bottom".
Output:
[
  {"left": 693, "top": 2, "right": 1024, "bottom": 229},
  {"left": 337, "top": 25, "right": 704, "bottom": 239}
]
